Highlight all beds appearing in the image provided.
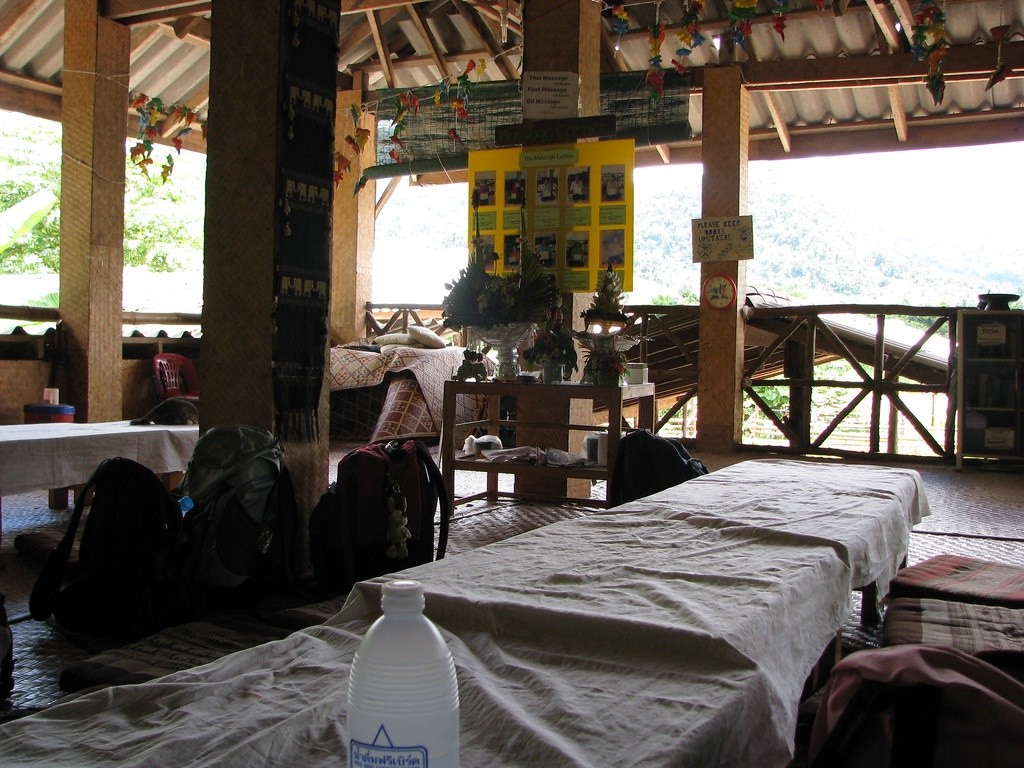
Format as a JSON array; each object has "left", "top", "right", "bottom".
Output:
[{"left": 329, "top": 325, "right": 495, "bottom": 445}]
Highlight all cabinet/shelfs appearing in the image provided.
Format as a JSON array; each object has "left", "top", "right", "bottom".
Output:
[
  {"left": 956, "top": 309, "right": 1024, "bottom": 472},
  {"left": 442, "top": 377, "right": 655, "bottom": 512}
]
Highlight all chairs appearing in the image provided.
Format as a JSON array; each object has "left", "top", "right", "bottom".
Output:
[{"left": 152, "top": 353, "right": 199, "bottom": 402}]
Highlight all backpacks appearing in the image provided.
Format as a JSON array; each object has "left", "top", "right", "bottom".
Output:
[
  {"left": 308, "top": 437, "right": 450, "bottom": 597},
  {"left": 28, "top": 458, "right": 189, "bottom": 657},
  {"left": 181, "top": 425, "right": 303, "bottom": 611},
  {"left": 603, "top": 425, "right": 711, "bottom": 509}
]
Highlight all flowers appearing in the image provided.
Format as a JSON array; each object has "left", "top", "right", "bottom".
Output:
[
  {"left": 440, "top": 235, "right": 558, "bottom": 333},
  {"left": 583, "top": 341, "right": 632, "bottom": 379},
  {"left": 524, "top": 322, "right": 576, "bottom": 379}
]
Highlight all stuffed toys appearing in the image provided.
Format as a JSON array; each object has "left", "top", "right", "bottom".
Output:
[{"left": 384, "top": 495, "right": 412, "bottom": 560}]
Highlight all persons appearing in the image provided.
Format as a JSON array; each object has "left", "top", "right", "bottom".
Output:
[{"left": 478, "top": 173, "right": 620, "bottom": 269}]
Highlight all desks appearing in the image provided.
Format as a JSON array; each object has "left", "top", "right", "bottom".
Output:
[
  {"left": 620, "top": 457, "right": 933, "bottom": 627},
  {"left": 0, "top": 615, "right": 794, "bottom": 768},
  {"left": 351, "top": 503, "right": 857, "bottom": 746},
  {"left": 0, "top": 419, "right": 201, "bottom": 534}
]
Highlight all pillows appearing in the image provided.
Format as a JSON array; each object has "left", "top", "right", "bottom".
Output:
[
  {"left": 372, "top": 333, "right": 416, "bottom": 345},
  {"left": 380, "top": 344, "right": 424, "bottom": 354},
  {"left": 408, "top": 325, "right": 445, "bottom": 348}
]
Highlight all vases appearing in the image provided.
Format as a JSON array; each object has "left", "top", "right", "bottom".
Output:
[
  {"left": 472, "top": 323, "right": 538, "bottom": 380},
  {"left": 542, "top": 362, "right": 564, "bottom": 383},
  {"left": 598, "top": 370, "right": 621, "bottom": 385}
]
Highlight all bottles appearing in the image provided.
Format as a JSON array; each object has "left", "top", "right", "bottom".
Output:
[{"left": 346, "top": 579, "right": 460, "bottom": 768}]
